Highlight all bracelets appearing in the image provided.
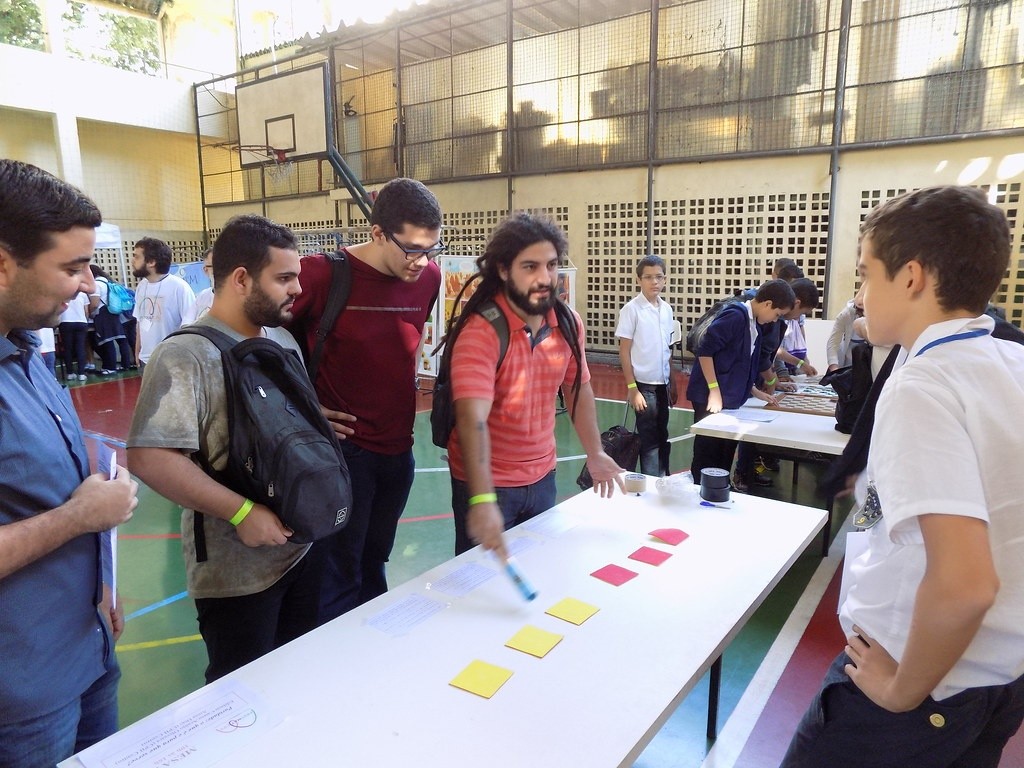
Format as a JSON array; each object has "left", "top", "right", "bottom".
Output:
[
  {"left": 708, "top": 381, "right": 719, "bottom": 389},
  {"left": 766, "top": 378, "right": 776, "bottom": 385},
  {"left": 627, "top": 382, "right": 638, "bottom": 388},
  {"left": 469, "top": 492, "right": 498, "bottom": 505},
  {"left": 230, "top": 498, "right": 254, "bottom": 526},
  {"left": 797, "top": 358, "right": 804, "bottom": 369}
]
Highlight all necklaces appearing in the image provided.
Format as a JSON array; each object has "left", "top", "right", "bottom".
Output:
[
  {"left": 913, "top": 327, "right": 990, "bottom": 358},
  {"left": 158, "top": 273, "right": 169, "bottom": 282}
]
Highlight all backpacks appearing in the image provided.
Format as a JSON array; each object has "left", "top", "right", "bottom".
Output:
[
  {"left": 95, "top": 279, "right": 133, "bottom": 314},
  {"left": 121, "top": 285, "right": 136, "bottom": 319},
  {"left": 686, "top": 293, "right": 755, "bottom": 356},
  {"left": 163, "top": 326, "right": 354, "bottom": 545},
  {"left": 429, "top": 271, "right": 581, "bottom": 451}
]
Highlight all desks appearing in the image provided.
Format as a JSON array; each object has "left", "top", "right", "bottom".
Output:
[
  {"left": 691, "top": 372, "right": 854, "bottom": 558},
  {"left": 49, "top": 470, "right": 830, "bottom": 768}
]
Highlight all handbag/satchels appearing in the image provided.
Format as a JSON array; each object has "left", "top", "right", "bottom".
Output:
[{"left": 819, "top": 363, "right": 858, "bottom": 434}]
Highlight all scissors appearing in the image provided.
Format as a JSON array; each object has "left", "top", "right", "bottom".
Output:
[{"left": 700, "top": 501, "right": 731, "bottom": 510}]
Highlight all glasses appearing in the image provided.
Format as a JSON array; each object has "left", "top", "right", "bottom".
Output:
[{"left": 381, "top": 227, "right": 446, "bottom": 260}]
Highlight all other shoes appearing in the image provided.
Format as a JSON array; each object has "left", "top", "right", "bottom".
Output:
[
  {"left": 755, "top": 452, "right": 779, "bottom": 470},
  {"left": 115, "top": 366, "right": 128, "bottom": 370},
  {"left": 745, "top": 468, "right": 772, "bottom": 485},
  {"left": 132, "top": 361, "right": 142, "bottom": 368},
  {"left": 68, "top": 372, "right": 77, "bottom": 379},
  {"left": 78, "top": 374, "right": 87, "bottom": 380},
  {"left": 95, "top": 368, "right": 117, "bottom": 376},
  {"left": 85, "top": 363, "right": 95, "bottom": 372},
  {"left": 733, "top": 472, "right": 750, "bottom": 494}
]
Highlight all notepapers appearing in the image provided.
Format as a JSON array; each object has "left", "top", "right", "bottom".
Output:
[
  {"left": 629, "top": 546, "right": 673, "bottom": 566},
  {"left": 648, "top": 528, "right": 689, "bottom": 546},
  {"left": 546, "top": 596, "right": 600, "bottom": 626},
  {"left": 449, "top": 659, "right": 514, "bottom": 699},
  {"left": 589, "top": 564, "right": 639, "bottom": 587},
  {"left": 505, "top": 624, "right": 564, "bottom": 658}
]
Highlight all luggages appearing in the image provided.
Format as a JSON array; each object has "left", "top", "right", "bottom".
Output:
[{"left": 577, "top": 399, "right": 641, "bottom": 491}]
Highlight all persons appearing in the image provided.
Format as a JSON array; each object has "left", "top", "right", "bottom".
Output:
[
  {"left": 447, "top": 213, "right": 628, "bottom": 560},
  {"left": 0, "top": 158, "right": 137, "bottom": 768},
  {"left": 828, "top": 283, "right": 1024, "bottom": 523},
  {"left": 779, "top": 184, "right": 1024, "bottom": 768},
  {"left": 29, "top": 264, "right": 136, "bottom": 381},
  {"left": 614, "top": 256, "right": 675, "bottom": 480},
  {"left": 195, "top": 244, "right": 218, "bottom": 327},
  {"left": 126, "top": 208, "right": 358, "bottom": 685},
  {"left": 730, "top": 276, "right": 819, "bottom": 492},
  {"left": 767, "top": 259, "right": 823, "bottom": 378},
  {"left": 278, "top": 176, "right": 442, "bottom": 608},
  {"left": 132, "top": 235, "right": 198, "bottom": 376},
  {"left": 686, "top": 280, "right": 797, "bottom": 494}
]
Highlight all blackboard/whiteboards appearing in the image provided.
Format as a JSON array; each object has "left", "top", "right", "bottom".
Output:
[{"left": 169, "top": 261, "right": 211, "bottom": 295}]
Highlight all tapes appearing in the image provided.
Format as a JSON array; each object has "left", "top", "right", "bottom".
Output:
[
  {"left": 699, "top": 484, "right": 731, "bottom": 502},
  {"left": 701, "top": 467, "right": 730, "bottom": 488},
  {"left": 626, "top": 473, "right": 647, "bottom": 491}
]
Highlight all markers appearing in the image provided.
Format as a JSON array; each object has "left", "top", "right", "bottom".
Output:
[{"left": 505, "top": 561, "right": 538, "bottom": 600}]
